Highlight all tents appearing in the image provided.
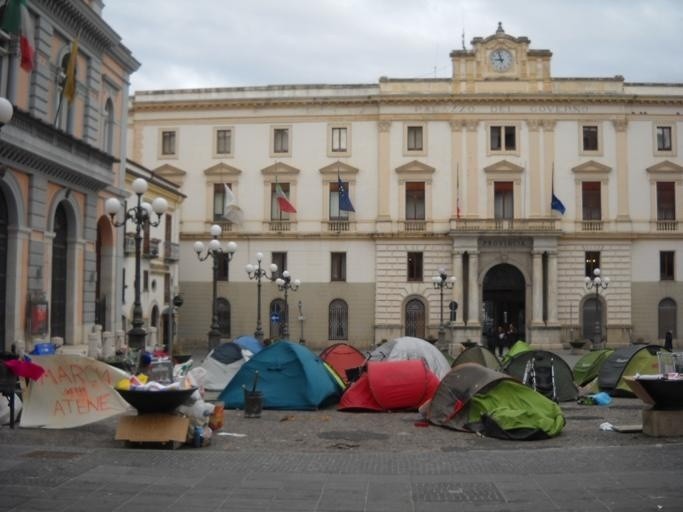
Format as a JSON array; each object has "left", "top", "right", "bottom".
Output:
[{"left": 200, "top": 335, "right": 682, "bottom": 443}]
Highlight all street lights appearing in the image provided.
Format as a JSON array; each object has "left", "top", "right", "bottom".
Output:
[
  {"left": 101, "top": 176, "right": 168, "bottom": 351},
  {"left": 193, "top": 221, "right": 236, "bottom": 355},
  {"left": 428, "top": 268, "right": 459, "bottom": 342},
  {"left": 272, "top": 269, "right": 302, "bottom": 343},
  {"left": 243, "top": 252, "right": 276, "bottom": 343},
  {"left": 582, "top": 264, "right": 615, "bottom": 351}
]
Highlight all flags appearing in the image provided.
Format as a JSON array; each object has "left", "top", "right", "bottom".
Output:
[
  {"left": 222, "top": 182, "right": 245, "bottom": 227},
  {"left": 276, "top": 184, "right": 297, "bottom": 214},
  {"left": 551, "top": 193, "right": 567, "bottom": 215},
  {"left": 338, "top": 177, "right": 356, "bottom": 213},
  {"left": 19, "top": 35, "right": 33, "bottom": 73},
  {"left": 64, "top": 32, "right": 78, "bottom": 105}
]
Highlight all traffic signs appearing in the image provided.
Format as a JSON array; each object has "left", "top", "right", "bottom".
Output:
[{"left": 0, "top": 96, "right": 15, "bottom": 130}]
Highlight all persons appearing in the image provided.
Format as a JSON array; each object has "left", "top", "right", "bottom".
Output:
[
  {"left": 504, "top": 323, "right": 518, "bottom": 349},
  {"left": 496, "top": 322, "right": 506, "bottom": 357}
]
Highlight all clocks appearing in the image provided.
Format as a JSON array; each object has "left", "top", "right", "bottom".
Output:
[{"left": 488, "top": 49, "right": 513, "bottom": 74}]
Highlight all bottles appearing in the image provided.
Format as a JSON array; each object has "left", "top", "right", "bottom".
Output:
[{"left": 193, "top": 426, "right": 203, "bottom": 448}]
